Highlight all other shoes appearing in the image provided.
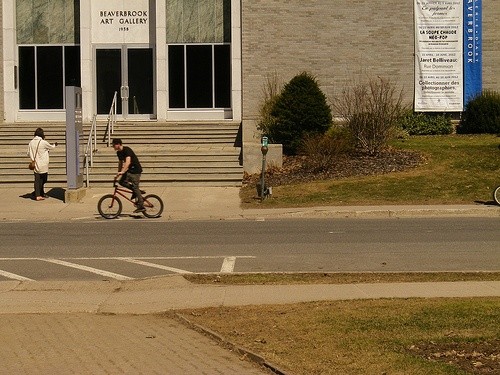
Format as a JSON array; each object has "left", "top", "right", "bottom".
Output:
[
  {"left": 135, "top": 207, "right": 146, "bottom": 214},
  {"left": 34, "top": 196, "right": 43, "bottom": 201}
]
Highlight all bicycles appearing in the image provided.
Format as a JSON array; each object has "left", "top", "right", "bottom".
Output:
[{"left": 97, "top": 174, "right": 164, "bottom": 219}]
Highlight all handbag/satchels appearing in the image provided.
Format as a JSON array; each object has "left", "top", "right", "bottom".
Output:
[{"left": 27, "top": 162, "right": 36, "bottom": 171}]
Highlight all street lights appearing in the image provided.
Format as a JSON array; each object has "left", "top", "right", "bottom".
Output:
[{"left": 261, "top": 136, "right": 268, "bottom": 202}]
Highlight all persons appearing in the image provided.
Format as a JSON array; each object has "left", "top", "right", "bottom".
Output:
[
  {"left": 112, "top": 139, "right": 146, "bottom": 213},
  {"left": 28, "top": 128, "right": 55, "bottom": 200}
]
